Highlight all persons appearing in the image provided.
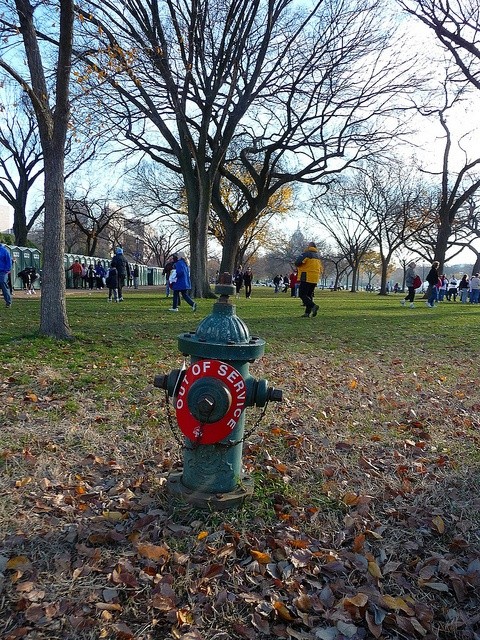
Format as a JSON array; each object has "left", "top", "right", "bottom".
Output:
[
  {"left": 471, "top": 273, "right": 480, "bottom": 305},
  {"left": 0, "top": 243, "right": 12, "bottom": 308},
  {"left": 273, "top": 275, "right": 280, "bottom": 293},
  {"left": 94, "top": 261, "right": 107, "bottom": 291},
  {"left": 295, "top": 242, "right": 324, "bottom": 318},
  {"left": 169, "top": 251, "right": 197, "bottom": 312},
  {"left": 127, "top": 264, "right": 133, "bottom": 288},
  {"left": 437, "top": 274, "right": 450, "bottom": 302},
  {"left": 66, "top": 260, "right": 83, "bottom": 289},
  {"left": 400, "top": 262, "right": 418, "bottom": 309},
  {"left": 278, "top": 274, "right": 284, "bottom": 292},
  {"left": 289, "top": 269, "right": 298, "bottom": 298},
  {"left": 161, "top": 255, "right": 177, "bottom": 299},
  {"left": 425, "top": 261, "right": 440, "bottom": 308},
  {"left": 17, "top": 267, "right": 39, "bottom": 295},
  {"left": 238, "top": 264, "right": 244, "bottom": 279},
  {"left": 469, "top": 276, "right": 476, "bottom": 304},
  {"left": 80, "top": 263, "right": 89, "bottom": 289},
  {"left": 387, "top": 282, "right": 391, "bottom": 294},
  {"left": 111, "top": 247, "right": 131, "bottom": 302},
  {"left": 0, "top": 256, "right": 14, "bottom": 295},
  {"left": 394, "top": 283, "right": 399, "bottom": 293},
  {"left": 105, "top": 261, "right": 119, "bottom": 303},
  {"left": 366, "top": 283, "right": 371, "bottom": 294},
  {"left": 329, "top": 281, "right": 335, "bottom": 288},
  {"left": 243, "top": 267, "right": 254, "bottom": 300},
  {"left": 282, "top": 274, "right": 289, "bottom": 293},
  {"left": 459, "top": 280, "right": 463, "bottom": 301},
  {"left": 235, "top": 269, "right": 243, "bottom": 300},
  {"left": 94, "top": 265, "right": 98, "bottom": 270},
  {"left": 86, "top": 264, "right": 96, "bottom": 290},
  {"left": 134, "top": 264, "right": 138, "bottom": 289},
  {"left": 336, "top": 283, "right": 342, "bottom": 292},
  {"left": 461, "top": 274, "right": 469, "bottom": 304},
  {"left": 448, "top": 274, "right": 458, "bottom": 302}
]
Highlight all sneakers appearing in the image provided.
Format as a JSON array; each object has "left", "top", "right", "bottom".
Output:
[
  {"left": 169, "top": 308, "right": 178, "bottom": 311},
  {"left": 120, "top": 298, "right": 123, "bottom": 302},
  {"left": 247, "top": 296, "right": 251, "bottom": 299},
  {"left": 193, "top": 302, "right": 196, "bottom": 312},
  {"left": 108, "top": 298, "right": 112, "bottom": 302},
  {"left": 25, "top": 290, "right": 31, "bottom": 295},
  {"left": 32, "top": 289, "right": 36, "bottom": 295},
  {"left": 400, "top": 301, "right": 405, "bottom": 308},
  {"left": 115, "top": 300, "right": 119, "bottom": 303},
  {"left": 430, "top": 305, "right": 437, "bottom": 308},
  {"left": 425, "top": 301, "right": 430, "bottom": 307},
  {"left": 410, "top": 303, "right": 414, "bottom": 308}
]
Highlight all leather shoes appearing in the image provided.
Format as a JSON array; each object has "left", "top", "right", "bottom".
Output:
[
  {"left": 301, "top": 313, "right": 309, "bottom": 317},
  {"left": 312, "top": 305, "right": 319, "bottom": 317}
]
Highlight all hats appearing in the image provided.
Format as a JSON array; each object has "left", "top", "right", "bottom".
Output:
[{"left": 117, "top": 249, "right": 123, "bottom": 254}]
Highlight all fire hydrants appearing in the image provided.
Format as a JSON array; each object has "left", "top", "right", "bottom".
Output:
[{"left": 151, "top": 268, "right": 287, "bottom": 509}]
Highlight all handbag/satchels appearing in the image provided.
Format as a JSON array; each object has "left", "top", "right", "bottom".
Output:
[
  {"left": 436, "top": 279, "right": 442, "bottom": 288},
  {"left": 413, "top": 275, "right": 422, "bottom": 289}
]
